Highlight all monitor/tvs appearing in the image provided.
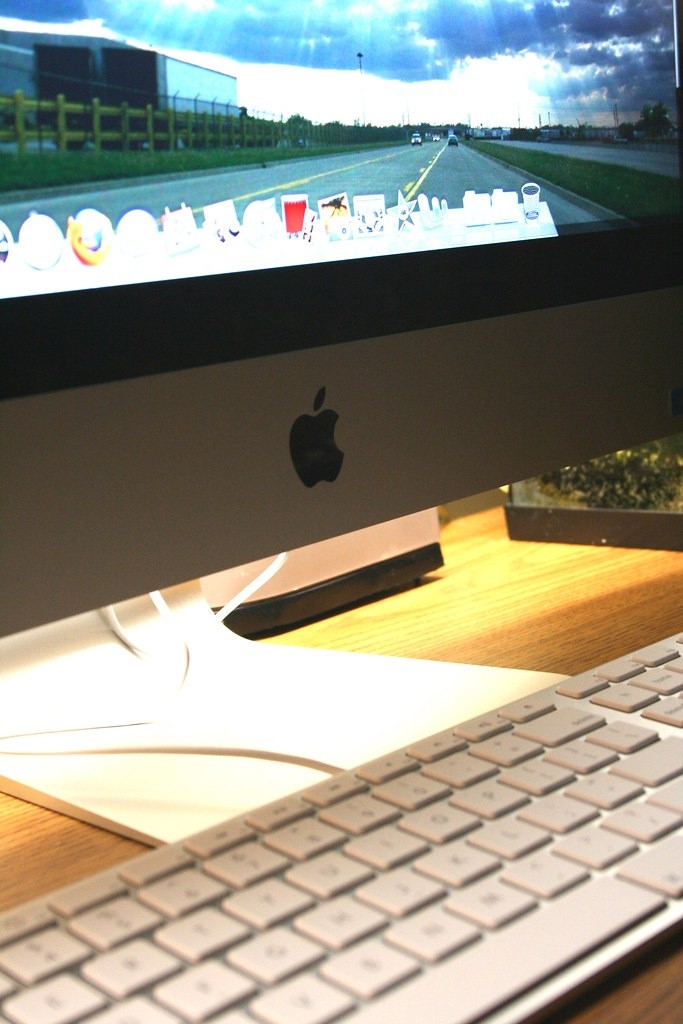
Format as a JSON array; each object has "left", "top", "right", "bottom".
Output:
[{"left": 0, "top": 0, "right": 683, "bottom": 847}]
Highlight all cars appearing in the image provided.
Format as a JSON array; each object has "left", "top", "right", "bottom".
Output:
[
  {"left": 448, "top": 134, "right": 459, "bottom": 147},
  {"left": 411, "top": 133, "right": 423, "bottom": 146},
  {"left": 432, "top": 134, "right": 440, "bottom": 142},
  {"left": 608, "top": 133, "right": 629, "bottom": 145}
]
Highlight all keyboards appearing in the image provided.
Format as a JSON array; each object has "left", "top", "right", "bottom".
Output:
[{"left": 1, "top": 632, "right": 683, "bottom": 1024}]
